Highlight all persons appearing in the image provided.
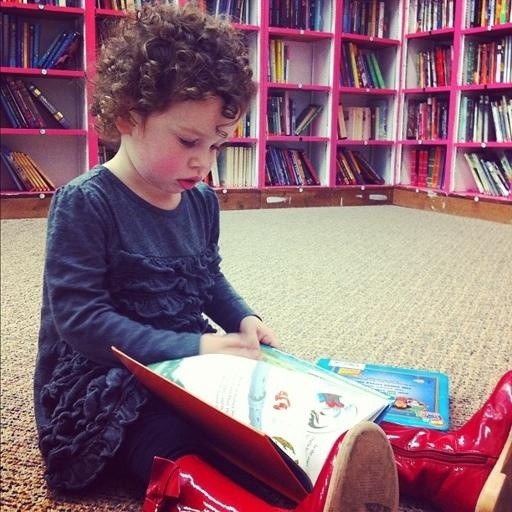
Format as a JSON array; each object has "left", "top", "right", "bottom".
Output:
[{"left": 34, "top": 0, "right": 512, "bottom": 512}]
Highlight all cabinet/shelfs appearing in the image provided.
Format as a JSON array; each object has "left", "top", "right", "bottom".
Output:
[
  {"left": 394, "top": 0, "right": 512, "bottom": 223},
  {"left": 0, "top": 0, "right": 405, "bottom": 220}
]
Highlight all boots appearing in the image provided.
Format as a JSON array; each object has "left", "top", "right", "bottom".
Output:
[
  {"left": 377, "top": 368, "right": 511, "bottom": 511},
  {"left": 142, "top": 417, "right": 400, "bottom": 512}
]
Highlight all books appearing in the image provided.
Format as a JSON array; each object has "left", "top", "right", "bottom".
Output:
[
  {"left": 1, "top": 0, "right": 512, "bottom": 205},
  {"left": 108, "top": 338, "right": 399, "bottom": 510}
]
